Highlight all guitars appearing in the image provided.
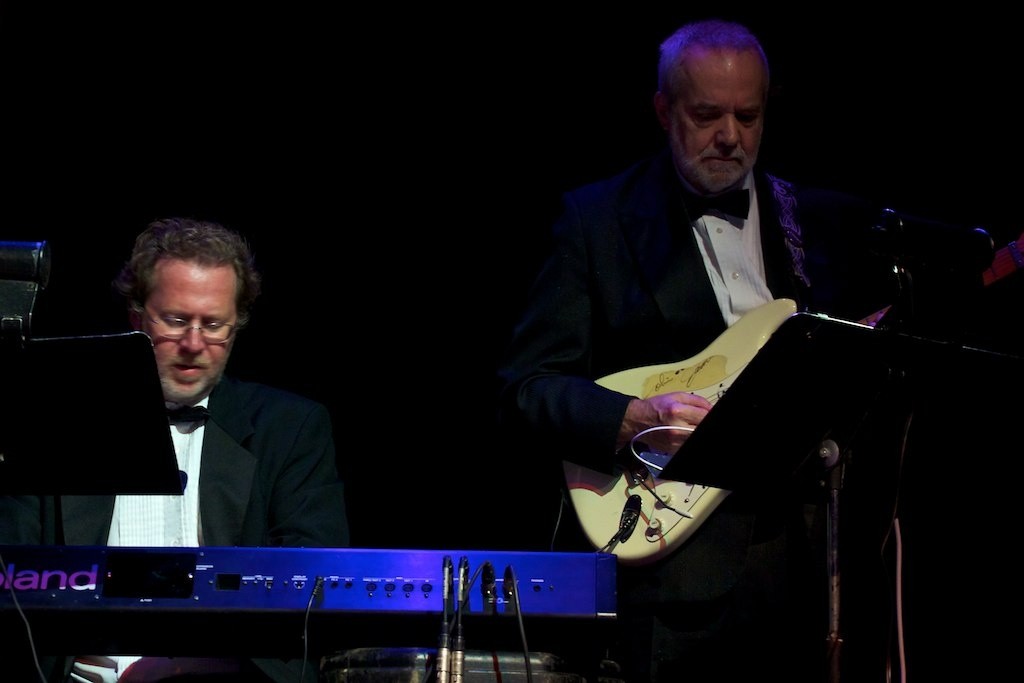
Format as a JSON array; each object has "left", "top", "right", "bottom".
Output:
[{"left": 559, "top": 233, "right": 1024, "bottom": 562}]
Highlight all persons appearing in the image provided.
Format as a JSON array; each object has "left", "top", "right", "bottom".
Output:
[
  {"left": 0, "top": 218, "right": 348, "bottom": 683},
  {"left": 496, "top": 21, "right": 825, "bottom": 683}
]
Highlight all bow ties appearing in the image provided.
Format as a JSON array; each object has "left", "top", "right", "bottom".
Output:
[
  {"left": 164, "top": 399, "right": 211, "bottom": 425},
  {"left": 671, "top": 189, "right": 750, "bottom": 222}
]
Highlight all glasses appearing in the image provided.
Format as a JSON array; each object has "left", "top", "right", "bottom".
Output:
[{"left": 144, "top": 306, "right": 239, "bottom": 344}]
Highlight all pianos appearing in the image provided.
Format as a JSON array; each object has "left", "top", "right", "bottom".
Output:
[{"left": 0, "top": 540, "right": 625, "bottom": 683}]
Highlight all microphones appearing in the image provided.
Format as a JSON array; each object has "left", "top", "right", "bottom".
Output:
[{"left": 869, "top": 207, "right": 909, "bottom": 320}]
